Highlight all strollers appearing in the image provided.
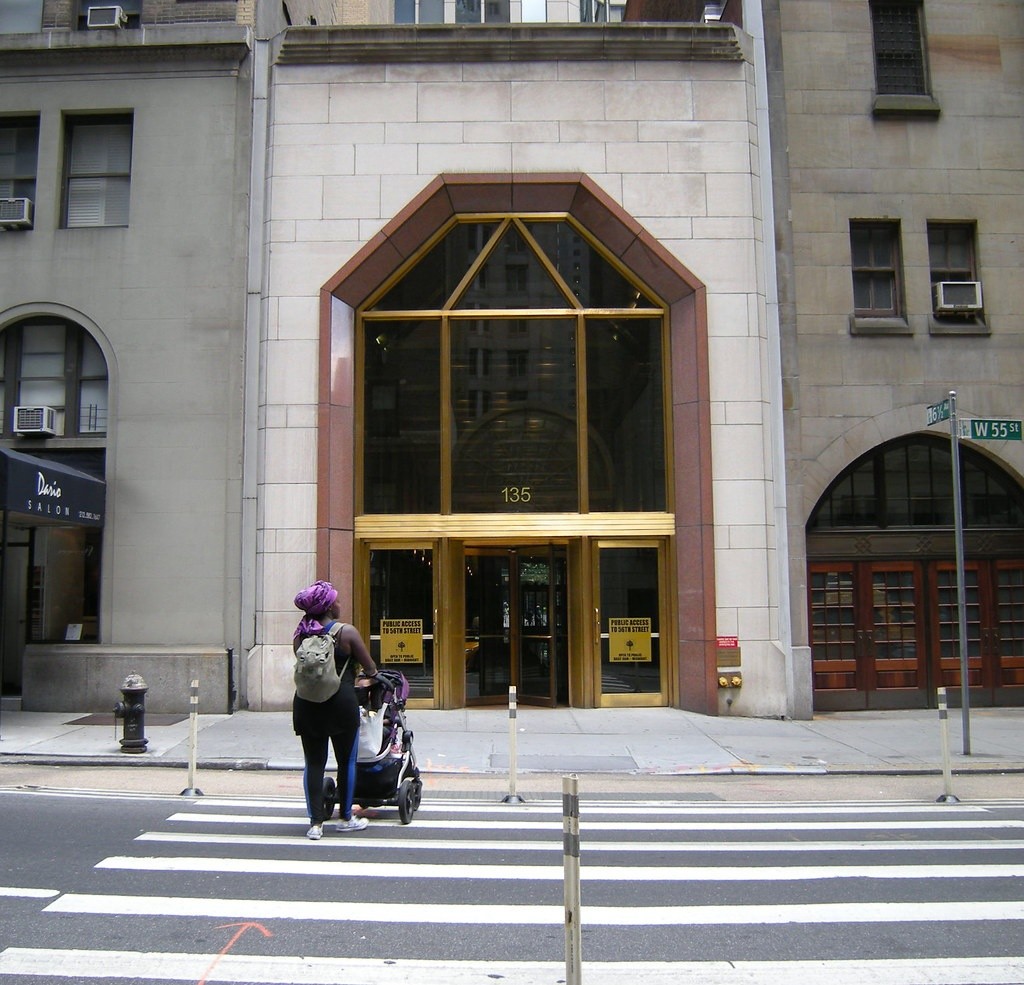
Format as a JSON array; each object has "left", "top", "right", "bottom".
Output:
[{"left": 317, "top": 666, "right": 423, "bottom": 822}]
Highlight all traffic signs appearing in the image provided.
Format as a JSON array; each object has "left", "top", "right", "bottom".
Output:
[
  {"left": 957, "top": 417, "right": 1022, "bottom": 440},
  {"left": 925, "top": 397, "right": 951, "bottom": 426}
]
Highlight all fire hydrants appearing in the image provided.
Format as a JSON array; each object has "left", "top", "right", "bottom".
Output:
[{"left": 112, "top": 668, "right": 150, "bottom": 754}]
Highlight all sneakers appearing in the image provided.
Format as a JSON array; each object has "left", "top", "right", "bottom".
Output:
[
  {"left": 307, "top": 825, "right": 324, "bottom": 839},
  {"left": 337, "top": 816, "right": 370, "bottom": 832}
]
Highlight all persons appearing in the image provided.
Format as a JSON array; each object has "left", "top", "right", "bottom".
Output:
[{"left": 289, "top": 580, "right": 379, "bottom": 840}]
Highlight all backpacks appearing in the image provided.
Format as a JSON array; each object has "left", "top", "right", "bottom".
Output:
[{"left": 293, "top": 621, "right": 350, "bottom": 704}]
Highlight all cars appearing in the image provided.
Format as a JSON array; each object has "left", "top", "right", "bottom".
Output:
[{"left": 463, "top": 616, "right": 482, "bottom": 672}]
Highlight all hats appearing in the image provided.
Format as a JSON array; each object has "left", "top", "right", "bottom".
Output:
[{"left": 293, "top": 581, "right": 338, "bottom": 616}]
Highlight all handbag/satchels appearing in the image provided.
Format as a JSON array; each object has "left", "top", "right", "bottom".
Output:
[{"left": 357, "top": 702, "right": 387, "bottom": 759}]
